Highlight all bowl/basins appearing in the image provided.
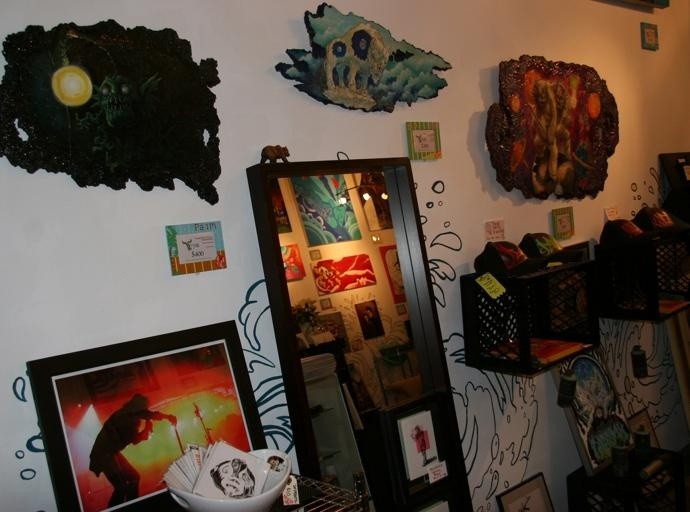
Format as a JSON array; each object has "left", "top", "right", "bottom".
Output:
[{"left": 167, "top": 450, "right": 292, "bottom": 509}]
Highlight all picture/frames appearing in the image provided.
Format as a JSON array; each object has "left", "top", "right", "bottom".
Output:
[
  {"left": 496, "top": 472, "right": 555, "bottom": 512},
  {"left": 551, "top": 207, "right": 576, "bottom": 241},
  {"left": 164, "top": 220, "right": 228, "bottom": 276},
  {"left": 640, "top": 20, "right": 659, "bottom": 50},
  {"left": 406, "top": 120, "right": 442, "bottom": 163},
  {"left": 22, "top": 316, "right": 269, "bottom": 512}
]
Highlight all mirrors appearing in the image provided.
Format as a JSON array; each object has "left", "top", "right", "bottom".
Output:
[{"left": 247, "top": 155, "right": 475, "bottom": 512}]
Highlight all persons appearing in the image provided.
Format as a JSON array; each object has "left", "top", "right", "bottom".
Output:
[{"left": 90, "top": 394, "right": 177, "bottom": 508}]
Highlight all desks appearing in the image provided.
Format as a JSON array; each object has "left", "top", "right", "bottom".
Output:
[{"left": 564, "top": 446, "right": 683, "bottom": 512}]
